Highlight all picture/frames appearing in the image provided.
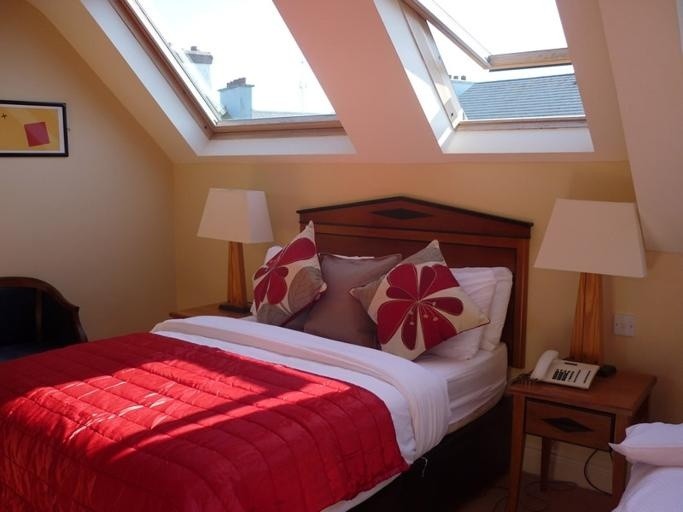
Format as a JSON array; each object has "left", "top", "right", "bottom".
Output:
[{"left": 0, "top": 99, "right": 69, "bottom": 158}]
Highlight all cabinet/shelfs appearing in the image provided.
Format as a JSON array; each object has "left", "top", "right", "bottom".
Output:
[{"left": 504, "top": 374, "right": 658, "bottom": 512}]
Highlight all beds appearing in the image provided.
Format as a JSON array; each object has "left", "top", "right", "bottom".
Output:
[
  {"left": 0, "top": 197, "right": 532, "bottom": 512},
  {"left": 614, "top": 462, "right": 683, "bottom": 512}
]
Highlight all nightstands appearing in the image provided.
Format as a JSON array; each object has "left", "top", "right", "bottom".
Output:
[{"left": 169, "top": 301, "right": 253, "bottom": 319}]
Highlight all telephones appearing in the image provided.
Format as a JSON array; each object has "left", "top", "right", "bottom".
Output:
[{"left": 530, "top": 351, "right": 600, "bottom": 389}]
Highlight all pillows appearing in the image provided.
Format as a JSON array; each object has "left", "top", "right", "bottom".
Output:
[
  {"left": 249, "top": 220, "right": 512, "bottom": 361},
  {"left": 608, "top": 422, "right": 683, "bottom": 467}
]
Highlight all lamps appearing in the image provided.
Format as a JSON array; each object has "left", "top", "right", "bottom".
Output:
[
  {"left": 533, "top": 198, "right": 647, "bottom": 367},
  {"left": 195, "top": 188, "right": 275, "bottom": 313}
]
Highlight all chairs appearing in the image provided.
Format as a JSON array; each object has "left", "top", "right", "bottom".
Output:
[{"left": 0, "top": 277, "right": 88, "bottom": 360}]
